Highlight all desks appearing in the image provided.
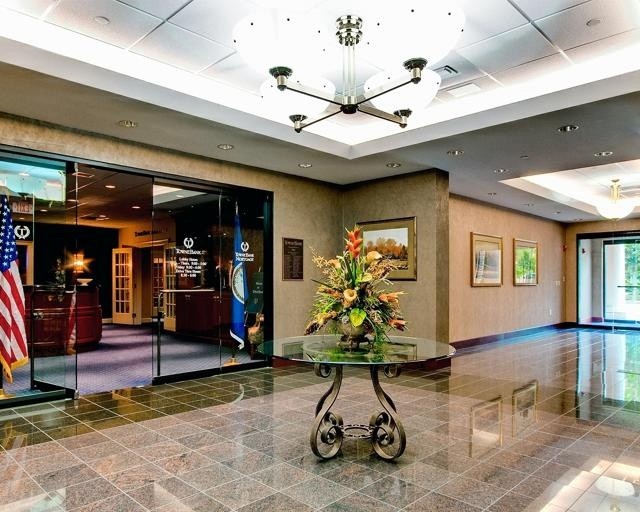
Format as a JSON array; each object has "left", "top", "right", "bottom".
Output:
[{"left": 257, "top": 334, "right": 456, "bottom": 461}]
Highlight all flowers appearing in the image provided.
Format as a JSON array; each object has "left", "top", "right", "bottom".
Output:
[{"left": 303, "top": 225, "right": 411, "bottom": 360}]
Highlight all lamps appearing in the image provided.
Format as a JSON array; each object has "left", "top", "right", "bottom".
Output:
[{"left": 232, "top": 5, "right": 460, "bottom": 134}]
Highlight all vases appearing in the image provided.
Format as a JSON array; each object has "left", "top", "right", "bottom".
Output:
[{"left": 340, "top": 320, "right": 371, "bottom": 350}]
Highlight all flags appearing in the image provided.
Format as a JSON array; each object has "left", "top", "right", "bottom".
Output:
[
  {"left": 0, "top": 194, "right": 30, "bottom": 383},
  {"left": 229, "top": 214, "right": 250, "bottom": 351}
]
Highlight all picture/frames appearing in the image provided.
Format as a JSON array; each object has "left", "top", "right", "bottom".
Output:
[
  {"left": 355, "top": 216, "right": 417, "bottom": 282},
  {"left": 468, "top": 378, "right": 540, "bottom": 462},
  {"left": 470, "top": 232, "right": 541, "bottom": 288}
]
